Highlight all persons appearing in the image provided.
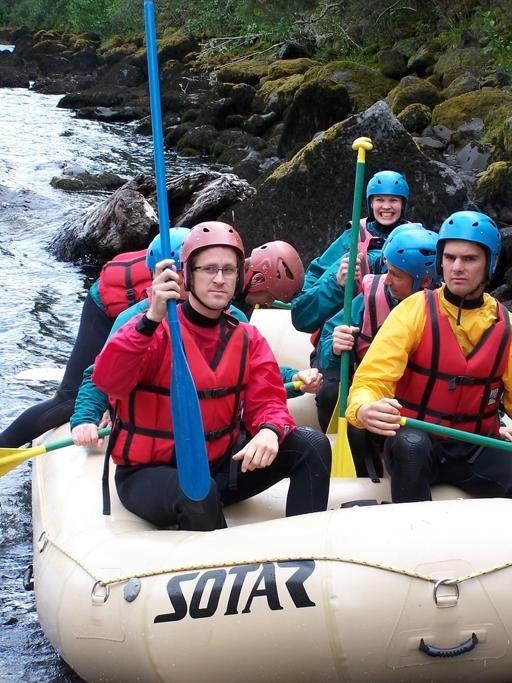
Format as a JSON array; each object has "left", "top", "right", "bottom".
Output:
[
  {"left": 301, "top": 170, "right": 409, "bottom": 292},
  {"left": 345, "top": 210, "right": 511, "bottom": 501},
  {"left": 0, "top": 227, "right": 190, "bottom": 448},
  {"left": 227, "top": 242, "right": 325, "bottom": 395},
  {"left": 92, "top": 221, "right": 333, "bottom": 531},
  {"left": 291, "top": 222, "right": 441, "bottom": 479}
]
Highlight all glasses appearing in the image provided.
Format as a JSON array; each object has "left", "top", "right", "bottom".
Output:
[{"left": 190, "top": 264, "right": 239, "bottom": 274}]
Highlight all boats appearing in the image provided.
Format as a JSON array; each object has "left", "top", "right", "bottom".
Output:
[{"left": 30, "top": 308, "right": 512, "bottom": 681}]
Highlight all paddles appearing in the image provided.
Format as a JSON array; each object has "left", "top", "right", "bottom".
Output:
[
  {"left": 144, "top": 0, "right": 210, "bottom": 502},
  {"left": 0, "top": 378, "right": 305, "bottom": 480},
  {"left": 324, "top": 137, "right": 373, "bottom": 478}
]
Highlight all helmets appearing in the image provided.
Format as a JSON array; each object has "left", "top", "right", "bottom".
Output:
[
  {"left": 180, "top": 221, "right": 245, "bottom": 293},
  {"left": 146, "top": 227, "right": 191, "bottom": 272},
  {"left": 243, "top": 241, "right": 304, "bottom": 304},
  {"left": 379, "top": 223, "right": 426, "bottom": 274},
  {"left": 383, "top": 229, "right": 443, "bottom": 293},
  {"left": 435, "top": 210, "right": 502, "bottom": 281},
  {"left": 366, "top": 170, "right": 409, "bottom": 219}
]
